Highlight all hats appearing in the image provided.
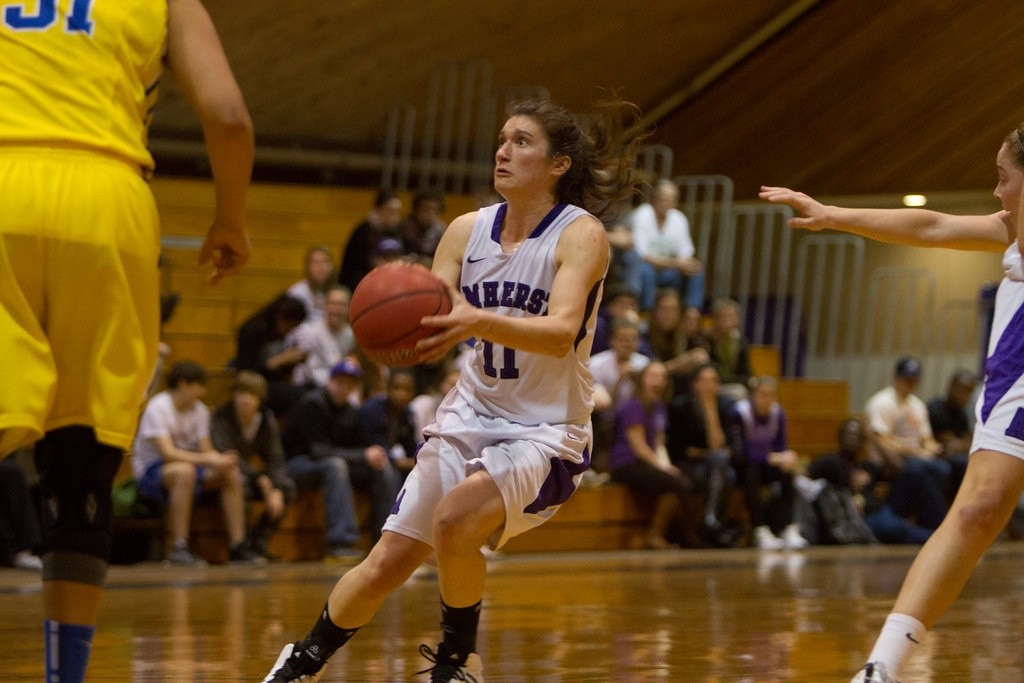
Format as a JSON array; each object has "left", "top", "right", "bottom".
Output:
[
  {"left": 334, "top": 361, "right": 361, "bottom": 380},
  {"left": 897, "top": 357, "right": 920, "bottom": 380}
]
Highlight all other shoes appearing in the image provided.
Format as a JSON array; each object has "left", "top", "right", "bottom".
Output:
[
  {"left": 252, "top": 541, "right": 281, "bottom": 564},
  {"left": 754, "top": 526, "right": 784, "bottom": 551},
  {"left": 227, "top": 540, "right": 266, "bottom": 565},
  {"left": 15, "top": 550, "right": 43, "bottom": 570},
  {"left": 851, "top": 662, "right": 902, "bottom": 683},
  {"left": 330, "top": 547, "right": 364, "bottom": 564},
  {"left": 779, "top": 523, "right": 807, "bottom": 551},
  {"left": 163, "top": 547, "right": 206, "bottom": 570}
]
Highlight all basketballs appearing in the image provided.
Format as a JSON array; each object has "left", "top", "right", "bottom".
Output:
[{"left": 348, "top": 260, "right": 453, "bottom": 369}]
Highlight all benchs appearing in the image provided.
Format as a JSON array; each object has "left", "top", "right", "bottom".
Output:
[{"left": 0, "top": 173, "right": 848, "bottom": 564}]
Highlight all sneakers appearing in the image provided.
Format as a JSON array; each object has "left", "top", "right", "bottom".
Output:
[
  {"left": 411, "top": 641, "right": 485, "bottom": 683},
  {"left": 262, "top": 642, "right": 327, "bottom": 683}
]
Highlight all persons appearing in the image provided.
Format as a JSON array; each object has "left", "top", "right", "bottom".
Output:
[
  {"left": 1, "top": 292, "right": 181, "bottom": 573},
  {"left": 992, "top": 121, "right": 1024, "bottom": 257},
  {"left": 233, "top": 189, "right": 469, "bottom": 575},
  {"left": 1, "top": 0, "right": 256, "bottom": 683},
  {"left": 758, "top": 184, "right": 1024, "bottom": 683},
  {"left": 807, "top": 356, "right": 1024, "bottom": 545},
  {"left": 585, "top": 179, "right": 830, "bottom": 550},
  {"left": 262, "top": 102, "right": 657, "bottom": 682},
  {"left": 132, "top": 360, "right": 269, "bottom": 569},
  {"left": 214, "top": 371, "right": 302, "bottom": 564}
]
